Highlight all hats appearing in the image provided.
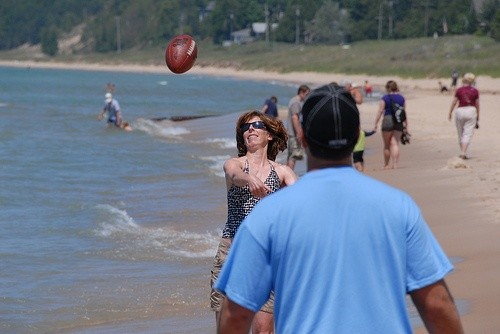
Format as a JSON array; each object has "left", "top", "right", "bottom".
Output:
[
  {"left": 300, "top": 82, "right": 359, "bottom": 156},
  {"left": 105, "top": 92, "right": 112, "bottom": 103}
]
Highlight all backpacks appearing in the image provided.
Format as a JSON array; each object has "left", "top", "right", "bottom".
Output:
[{"left": 390, "top": 100, "right": 406, "bottom": 125}]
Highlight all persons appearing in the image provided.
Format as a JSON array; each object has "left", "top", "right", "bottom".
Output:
[
  {"left": 260, "top": 72, "right": 479, "bottom": 175},
  {"left": 215, "top": 83, "right": 468, "bottom": 334},
  {"left": 210, "top": 111, "right": 299, "bottom": 334},
  {"left": 97, "top": 93, "right": 126, "bottom": 128}
]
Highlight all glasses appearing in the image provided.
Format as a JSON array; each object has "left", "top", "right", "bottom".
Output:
[{"left": 240, "top": 121, "right": 266, "bottom": 133}]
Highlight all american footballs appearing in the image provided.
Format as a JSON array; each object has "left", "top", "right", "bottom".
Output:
[{"left": 165, "top": 35, "right": 198, "bottom": 75}]
